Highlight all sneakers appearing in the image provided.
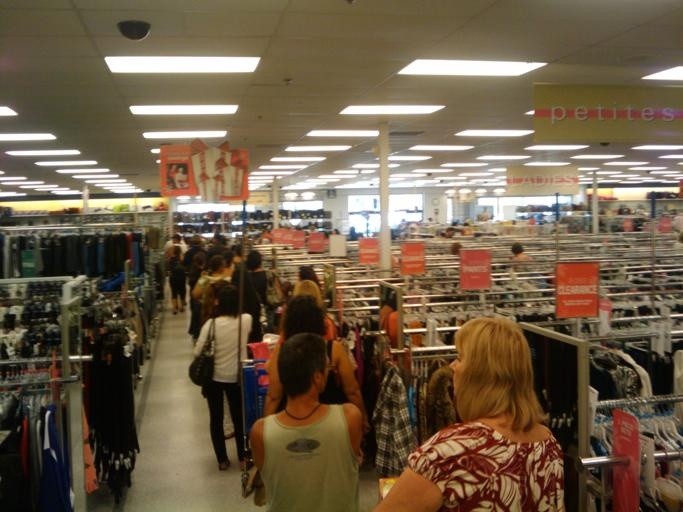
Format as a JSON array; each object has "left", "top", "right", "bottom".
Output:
[{"left": 218, "top": 427, "right": 248, "bottom": 471}]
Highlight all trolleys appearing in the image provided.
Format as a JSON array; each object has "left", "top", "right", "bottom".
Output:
[{"left": 229, "top": 353, "right": 273, "bottom": 498}]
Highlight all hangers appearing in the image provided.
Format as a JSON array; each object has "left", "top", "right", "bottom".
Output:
[
  {"left": 2, "top": 355, "right": 64, "bottom": 407},
  {"left": 587, "top": 332, "right": 668, "bottom": 368},
  {"left": 400, "top": 356, "right": 457, "bottom": 396},
  {"left": 335, "top": 309, "right": 510, "bottom": 339},
  {"left": 595, "top": 395, "right": 682, "bottom": 508}
]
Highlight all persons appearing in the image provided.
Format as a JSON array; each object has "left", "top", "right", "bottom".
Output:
[
  {"left": 162, "top": 228, "right": 337, "bottom": 474},
  {"left": 264, "top": 294, "right": 371, "bottom": 434},
  {"left": 451, "top": 242, "right": 463, "bottom": 256},
  {"left": 441, "top": 227, "right": 456, "bottom": 238},
  {"left": 168, "top": 164, "right": 178, "bottom": 189},
  {"left": 252, "top": 334, "right": 363, "bottom": 511},
  {"left": 371, "top": 316, "right": 567, "bottom": 511},
  {"left": 509, "top": 242, "right": 536, "bottom": 273},
  {"left": 173, "top": 166, "right": 188, "bottom": 188},
  {"left": 348, "top": 226, "right": 357, "bottom": 241}
]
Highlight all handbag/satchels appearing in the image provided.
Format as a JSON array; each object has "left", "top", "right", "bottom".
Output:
[
  {"left": 263, "top": 272, "right": 284, "bottom": 307},
  {"left": 188, "top": 338, "right": 217, "bottom": 385}
]
